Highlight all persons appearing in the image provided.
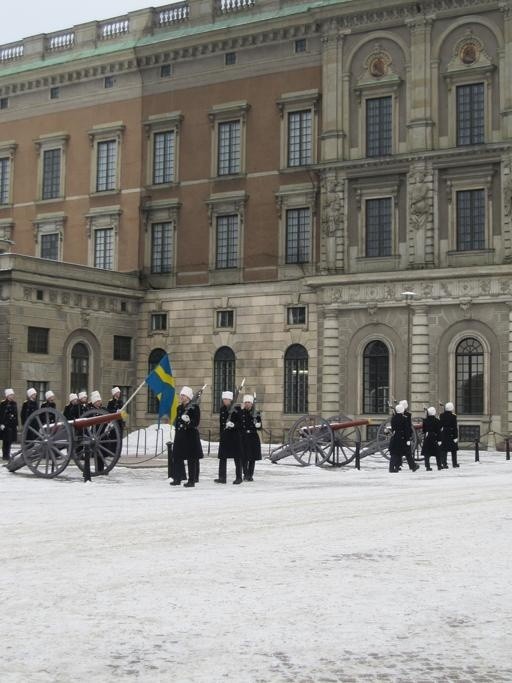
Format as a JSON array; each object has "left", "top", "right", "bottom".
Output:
[
  {"left": 214, "top": 391, "right": 242, "bottom": 484},
  {"left": 107, "top": 386, "right": 127, "bottom": 458},
  {"left": 0, "top": 388, "right": 19, "bottom": 460},
  {"left": 170, "top": 386, "right": 200, "bottom": 486},
  {"left": 421, "top": 406, "right": 443, "bottom": 471},
  {"left": 439, "top": 402, "right": 460, "bottom": 469},
  {"left": 39, "top": 390, "right": 57, "bottom": 459},
  {"left": 226, "top": 394, "right": 263, "bottom": 481},
  {"left": 194, "top": 440, "right": 204, "bottom": 482},
  {"left": 62, "top": 390, "right": 109, "bottom": 475},
  {"left": 20, "top": 387, "right": 39, "bottom": 449},
  {"left": 389, "top": 405, "right": 419, "bottom": 472},
  {"left": 394, "top": 401, "right": 418, "bottom": 470}
]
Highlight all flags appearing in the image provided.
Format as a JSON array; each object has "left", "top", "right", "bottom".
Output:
[{"left": 144, "top": 354, "right": 178, "bottom": 428}]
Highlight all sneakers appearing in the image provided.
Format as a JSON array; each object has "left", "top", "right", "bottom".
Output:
[
  {"left": 184, "top": 483, "right": 194, "bottom": 487},
  {"left": 233, "top": 479, "right": 243, "bottom": 484},
  {"left": 214, "top": 479, "right": 226, "bottom": 483},
  {"left": 170, "top": 481, "right": 180, "bottom": 485}
]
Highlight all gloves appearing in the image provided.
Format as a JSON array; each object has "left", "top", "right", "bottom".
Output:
[
  {"left": 182, "top": 415, "right": 190, "bottom": 422},
  {"left": 438, "top": 442, "right": 442, "bottom": 446},
  {"left": 454, "top": 439, "right": 458, "bottom": 443},
  {"left": 254, "top": 422, "right": 262, "bottom": 428},
  {"left": 226, "top": 421, "right": 234, "bottom": 427},
  {"left": 406, "top": 441, "right": 411, "bottom": 445},
  {"left": 1, "top": 425, "right": 5, "bottom": 431}
]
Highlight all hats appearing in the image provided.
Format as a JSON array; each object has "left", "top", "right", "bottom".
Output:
[
  {"left": 5, "top": 388, "right": 14, "bottom": 397},
  {"left": 243, "top": 395, "right": 254, "bottom": 404},
  {"left": 396, "top": 404, "right": 404, "bottom": 413},
  {"left": 445, "top": 402, "right": 454, "bottom": 411},
  {"left": 27, "top": 388, "right": 37, "bottom": 398},
  {"left": 180, "top": 386, "right": 193, "bottom": 400},
  {"left": 45, "top": 391, "right": 55, "bottom": 400},
  {"left": 69, "top": 393, "right": 78, "bottom": 401},
  {"left": 78, "top": 392, "right": 88, "bottom": 399},
  {"left": 222, "top": 391, "right": 233, "bottom": 400},
  {"left": 91, "top": 391, "right": 101, "bottom": 404},
  {"left": 112, "top": 387, "right": 120, "bottom": 395},
  {"left": 399, "top": 400, "right": 408, "bottom": 409},
  {"left": 428, "top": 407, "right": 436, "bottom": 416}
]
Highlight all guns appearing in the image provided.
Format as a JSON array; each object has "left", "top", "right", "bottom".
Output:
[
  {"left": 387, "top": 401, "right": 396, "bottom": 415},
  {"left": 253, "top": 392, "right": 259, "bottom": 426},
  {"left": 222, "top": 377, "right": 246, "bottom": 428},
  {"left": 179, "top": 383, "right": 207, "bottom": 425},
  {"left": 438, "top": 401, "right": 445, "bottom": 412},
  {"left": 422, "top": 402, "right": 427, "bottom": 417},
  {"left": 392, "top": 397, "right": 399, "bottom": 405}
]
